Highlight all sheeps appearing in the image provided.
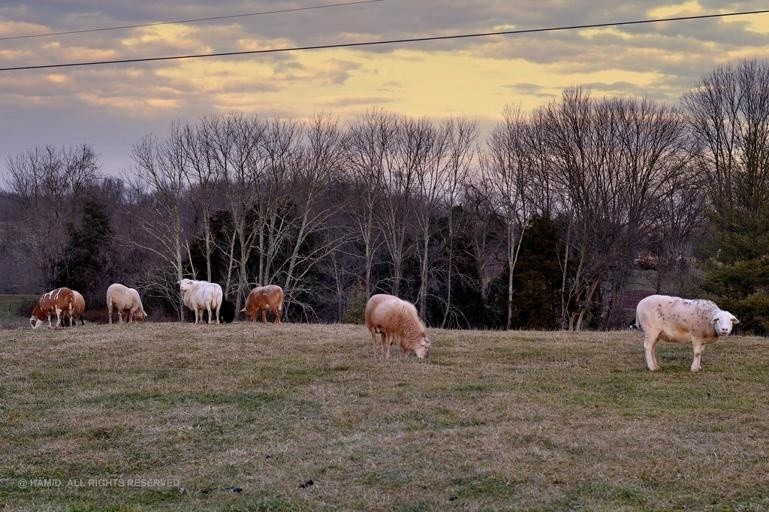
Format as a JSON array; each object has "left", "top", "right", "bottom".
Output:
[
  {"left": 176, "top": 278, "right": 224, "bottom": 325},
  {"left": 29, "top": 287, "right": 74, "bottom": 328},
  {"left": 61, "top": 288, "right": 86, "bottom": 328},
  {"left": 364, "top": 292, "right": 433, "bottom": 362},
  {"left": 105, "top": 282, "right": 148, "bottom": 324},
  {"left": 631, "top": 293, "right": 740, "bottom": 372},
  {"left": 239, "top": 285, "right": 288, "bottom": 324}
]
